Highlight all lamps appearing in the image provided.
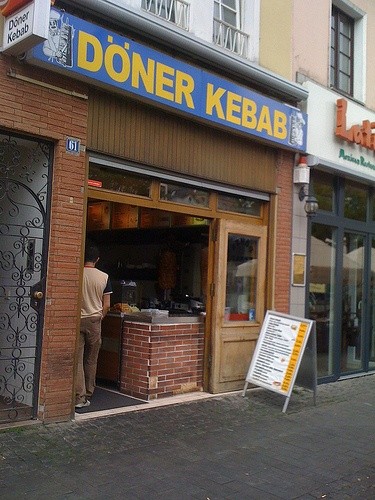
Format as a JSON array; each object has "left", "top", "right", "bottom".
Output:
[{"left": 299, "top": 185, "right": 320, "bottom": 217}]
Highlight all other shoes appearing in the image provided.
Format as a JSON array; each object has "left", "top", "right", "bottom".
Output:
[{"left": 75, "top": 399, "right": 92, "bottom": 407}]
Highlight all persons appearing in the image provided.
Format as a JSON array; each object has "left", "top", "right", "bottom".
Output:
[{"left": 74, "top": 244, "right": 114, "bottom": 408}]
